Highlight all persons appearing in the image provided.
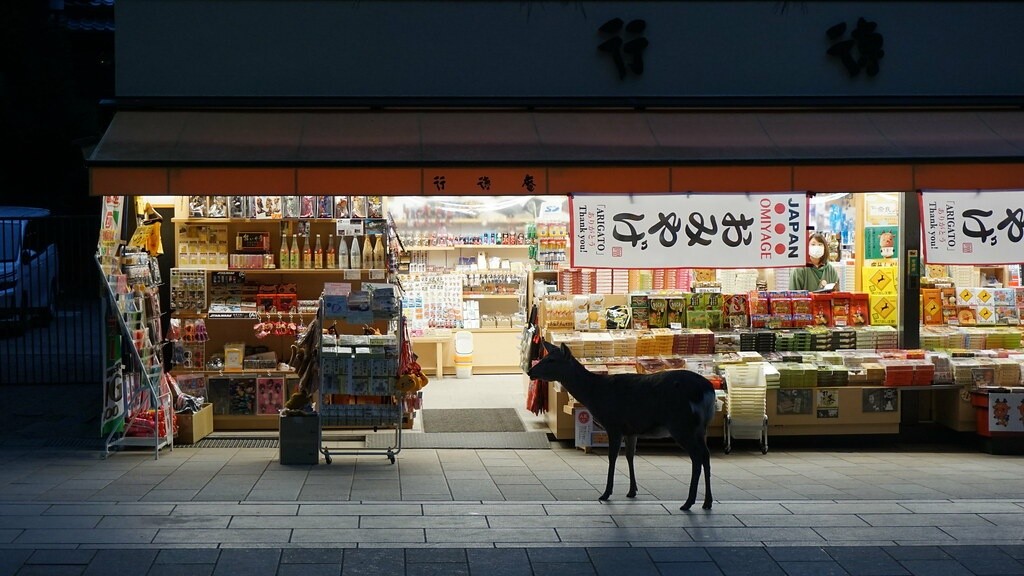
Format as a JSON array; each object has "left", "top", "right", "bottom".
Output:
[{"left": 789, "top": 231, "right": 841, "bottom": 292}]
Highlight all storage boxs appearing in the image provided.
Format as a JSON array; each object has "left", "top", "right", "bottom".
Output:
[
  {"left": 279, "top": 413, "right": 321, "bottom": 465},
  {"left": 725, "top": 366, "right": 766, "bottom": 439},
  {"left": 175, "top": 403, "right": 213, "bottom": 443},
  {"left": 453, "top": 331, "right": 473, "bottom": 379}
]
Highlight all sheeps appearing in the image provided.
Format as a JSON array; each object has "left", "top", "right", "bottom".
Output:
[{"left": 527, "top": 340, "right": 717, "bottom": 511}]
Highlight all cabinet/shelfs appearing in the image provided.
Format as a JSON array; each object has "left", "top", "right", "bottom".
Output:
[
  {"left": 919, "top": 295, "right": 1024, "bottom": 432},
  {"left": 538, "top": 294, "right": 901, "bottom": 439},
  {"left": 390, "top": 246, "right": 456, "bottom": 378},
  {"left": 171, "top": 196, "right": 388, "bottom": 429},
  {"left": 95, "top": 254, "right": 173, "bottom": 460},
  {"left": 454, "top": 239, "right": 530, "bottom": 375}
]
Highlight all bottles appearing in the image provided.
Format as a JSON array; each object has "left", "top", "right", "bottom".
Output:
[
  {"left": 302, "top": 237, "right": 311, "bottom": 269},
  {"left": 339, "top": 236, "right": 348, "bottom": 269},
  {"left": 326, "top": 234, "right": 335, "bottom": 269},
  {"left": 373, "top": 235, "right": 384, "bottom": 269},
  {"left": 290, "top": 234, "right": 299, "bottom": 269},
  {"left": 351, "top": 235, "right": 360, "bottom": 269},
  {"left": 363, "top": 235, "right": 373, "bottom": 269},
  {"left": 314, "top": 234, "right": 322, "bottom": 269},
  {"left": 279, "top": 233, "right": 289, "bottom": 269}
]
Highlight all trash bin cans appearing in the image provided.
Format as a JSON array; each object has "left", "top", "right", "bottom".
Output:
[{"left": 278, "top": 408, "right": 321, "bottom": 466}]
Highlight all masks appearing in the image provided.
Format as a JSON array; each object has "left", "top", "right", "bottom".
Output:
[{"left": 808, "top": 246, "right": 825, "bottom": 259}]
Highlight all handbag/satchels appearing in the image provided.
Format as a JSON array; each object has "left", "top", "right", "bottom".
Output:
[
  {"left": 395, "top": 322, "right": 428, "bottom": 392},
  {"left": 518, "top": 304, "right": 549, "bottom": 416}
]
